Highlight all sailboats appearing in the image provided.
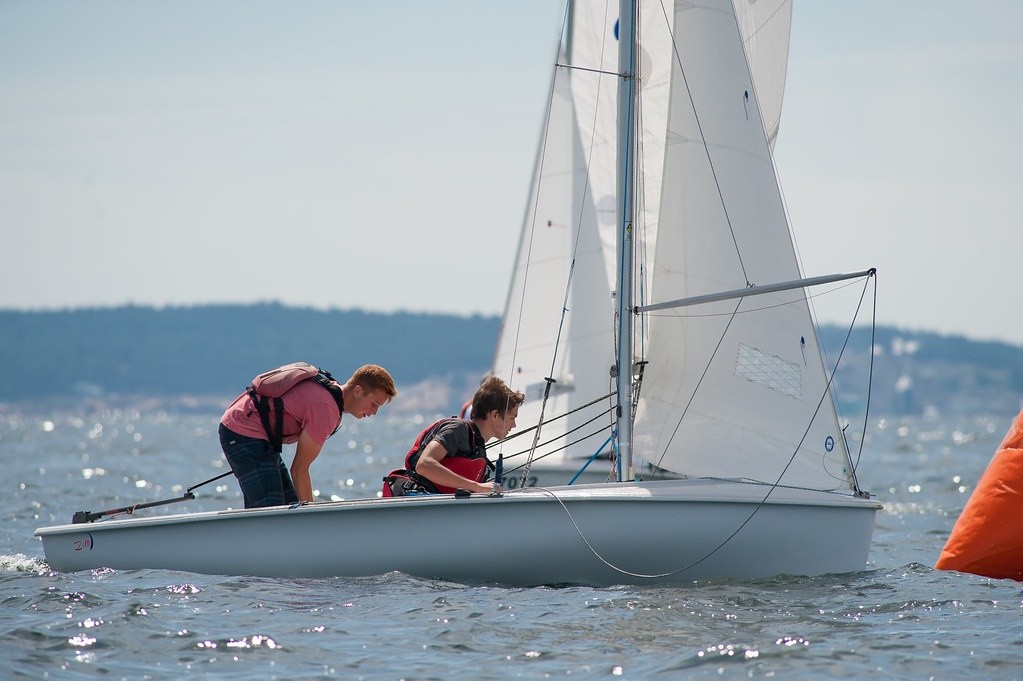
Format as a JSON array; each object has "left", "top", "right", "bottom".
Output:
[{"left": 35, "top": 1, "right": 890, "bottom": 572}]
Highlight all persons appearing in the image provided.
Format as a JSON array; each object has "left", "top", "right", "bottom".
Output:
[
  {"left": 217, "top": 361, "right": 396, "bottom": 508},
  {"left": 383, "top": 375, "right": 524, "bottom": 497}
]
